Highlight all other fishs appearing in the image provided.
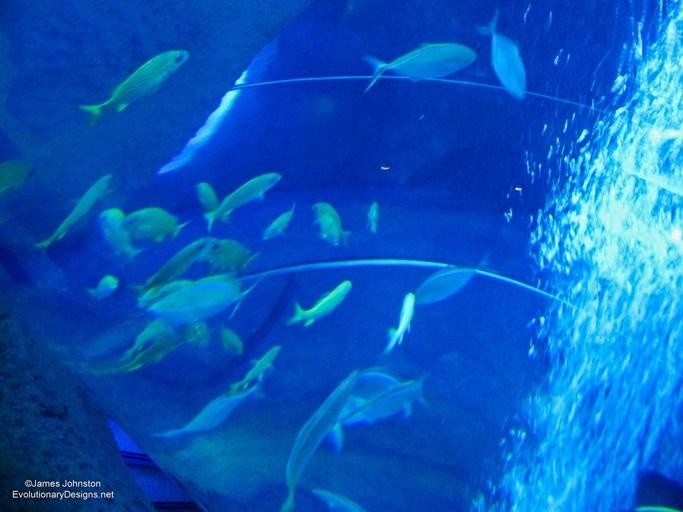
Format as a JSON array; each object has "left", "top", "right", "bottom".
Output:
[{"left": 84, "top": 171, "right": 379, "bottom": 442}]
